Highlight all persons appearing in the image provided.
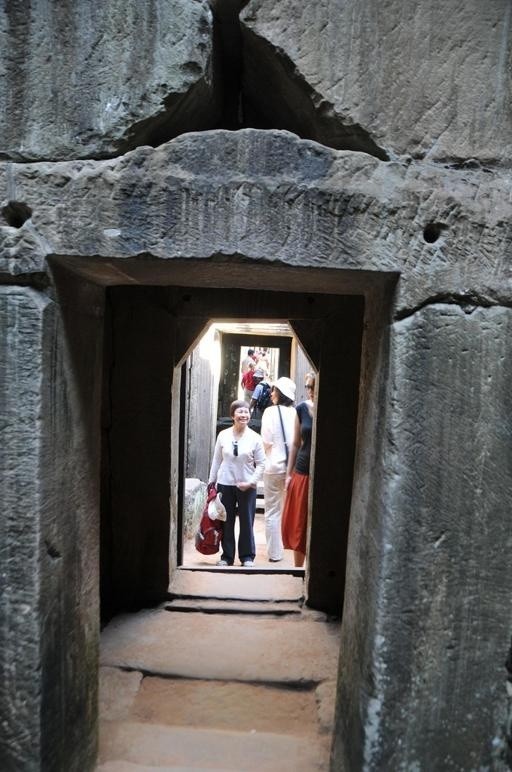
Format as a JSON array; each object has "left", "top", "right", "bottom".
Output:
[
  {"left": 281, "top": 372, "right": 316, "bottom": 567},
  {"left": 241, "top": 347, "right": 272, "bottom": 421},
  {"left": 208, "top": 400, "right": 267, "bottom": 568},
  {"left": 258, "top": 376, "right": 298, "bottom": 563}
]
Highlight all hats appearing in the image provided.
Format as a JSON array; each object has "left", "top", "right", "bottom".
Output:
[
  {"left": 271, "top": 377, "right": 296, "bottom": 402},
  {"left": 253, "top": 369, "right": 265, "bottom": 378},
  {"left": 208, "top": 492, "right": 227, "bottom": 522}
]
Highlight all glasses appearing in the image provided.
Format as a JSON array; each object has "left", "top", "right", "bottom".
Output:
[
  {"left": 233, "top": 440, "right": 238, "bottom": 457},
  {"left": 304, "top": 384, "right": 314, "bottom": 390}
]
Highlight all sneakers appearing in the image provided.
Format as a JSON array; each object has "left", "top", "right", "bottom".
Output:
[
  {"left": 215, "top": 559, "right": 228, "bottom": 566},
  {"left": 241, "top": 561, "right": 256, "bottom": 567}
]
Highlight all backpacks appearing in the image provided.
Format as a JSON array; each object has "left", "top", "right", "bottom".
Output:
[
  {"left": 195, "top": 483, "right": 223, "bottom": 555},
  {"left": 257, "top": 383, "right": 273, "bottom": 412}
]
